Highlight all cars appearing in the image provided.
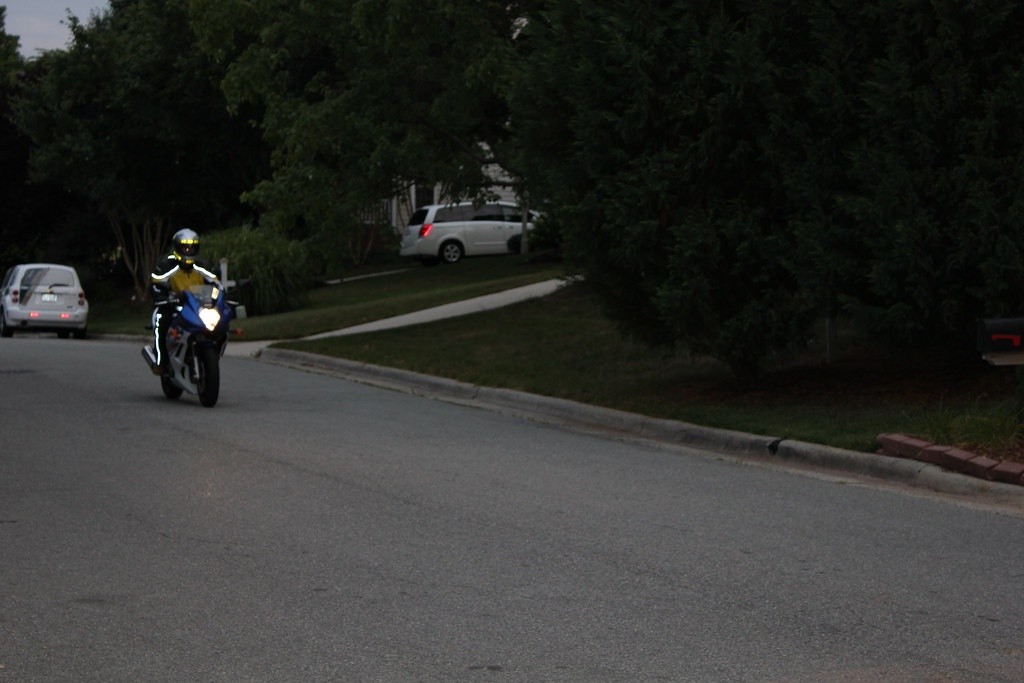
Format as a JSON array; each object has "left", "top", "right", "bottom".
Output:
[{"left": 0, "top": 262, "right": 90, "bottom": 339}]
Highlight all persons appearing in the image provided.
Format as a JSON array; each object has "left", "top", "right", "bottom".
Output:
[{"left": 150, "top": 227, "right": 230, "bottom": 375}]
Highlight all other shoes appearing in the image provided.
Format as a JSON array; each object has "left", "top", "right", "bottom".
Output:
[{"left": 153, "top": 363, "right": 176, "bottom": 378}]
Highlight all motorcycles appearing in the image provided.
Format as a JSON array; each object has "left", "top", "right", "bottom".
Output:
[{"left": 141, "top": 281, "right": 244, "bottom": 410}]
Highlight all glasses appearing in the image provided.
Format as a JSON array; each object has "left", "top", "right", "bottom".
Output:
[{"left": 180, "top": 246, "right": 195, "bottom": 253}]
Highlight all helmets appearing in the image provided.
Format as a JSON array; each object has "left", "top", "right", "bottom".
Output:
[{"left": 172, "top": 229, "right": 200, "bottom": 265}]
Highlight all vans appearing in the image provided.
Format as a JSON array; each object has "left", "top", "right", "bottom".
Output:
[{"left": 397, "top": 200, "right": 542, "bottom": 267}]
